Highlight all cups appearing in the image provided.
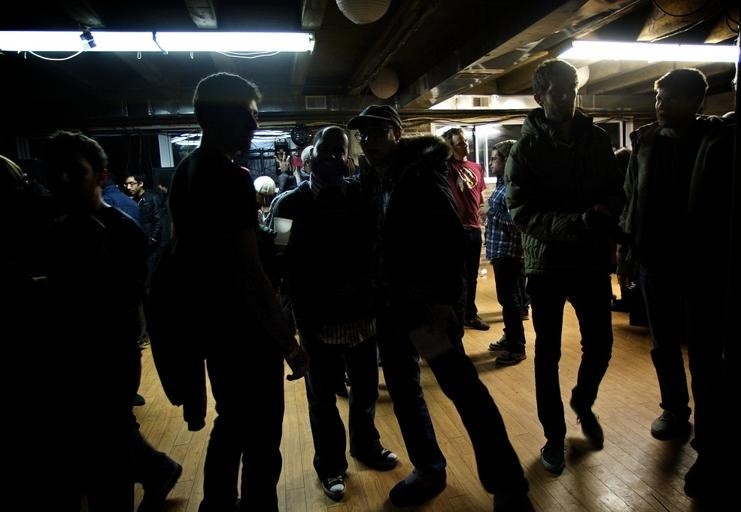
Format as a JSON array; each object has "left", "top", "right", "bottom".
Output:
[{"left": 274, "top": 216, "right": 293, "bottom": 245}]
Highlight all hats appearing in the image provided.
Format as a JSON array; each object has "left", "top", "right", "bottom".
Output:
[
  {"left": 301, "top": 145, "right": 315, "bottom": 163},
  {"left": 254, "top": 176, "right": 279, "bottom": 196},
  {"left": 493, "top": 139, "right": 515, "bottom": 161},
  {"left": 346, "top": 105, "right": 402, "bottom": 142}
]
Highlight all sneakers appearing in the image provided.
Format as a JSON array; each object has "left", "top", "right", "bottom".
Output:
[
  {"left": 137, "top": 457, "right": 182, "bottom": 511},
  {"left": 490, "top": 335, "right": 510, "bottom": 350},
  {"left": 139, "top": 338, "right": 151, "bottom": 348},
  {"left": 494, "top": 491, "right": 535, "bottom": 511},
  {"left": 133, "top": 394, "right": 145, "bottom": 405},
  {"left": 463, "top": 316, "right": 490, "bottom": 330},
  {"left": 388, "top": 469, "right": 446, "bottom": 508},
  {"left": 321, "top": 472, "right": 347, "bottom": 500},
  {"left": 370, "top": 448, "right": 398, "bottom": 471},
  {"left": 496, "top": 345, "right": 526, "bottom": 365},
  {"left": 542, "top": 405, "right": 691, "bottom": 472}
]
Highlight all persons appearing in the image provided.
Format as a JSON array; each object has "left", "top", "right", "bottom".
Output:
[
  {"left": 1, "top": 130, "right": 182, "bottom": 511},
  {"left": 166, "top": 72, "right": 533, "bottom": 511},
  {"left": 484, "top": 61, "right": 739, "bottom": 510}
]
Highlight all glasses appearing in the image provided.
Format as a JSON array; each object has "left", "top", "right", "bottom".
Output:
[
  {"left": 124, "top": 182, "right": 139, "bottom": 188},
  {"left": 489, "top": 155, "right": 504, "bottom": 162},
  {"left": 355, "top": 126, "right": 396, "bottom": 142}
]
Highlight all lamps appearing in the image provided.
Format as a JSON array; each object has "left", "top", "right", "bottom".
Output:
[
  {"left": 0, "top": 28, "right": 317, "bottom": 55},
  {"left": 550, "top": 38, "right": 741, "bottom": 63}
]
